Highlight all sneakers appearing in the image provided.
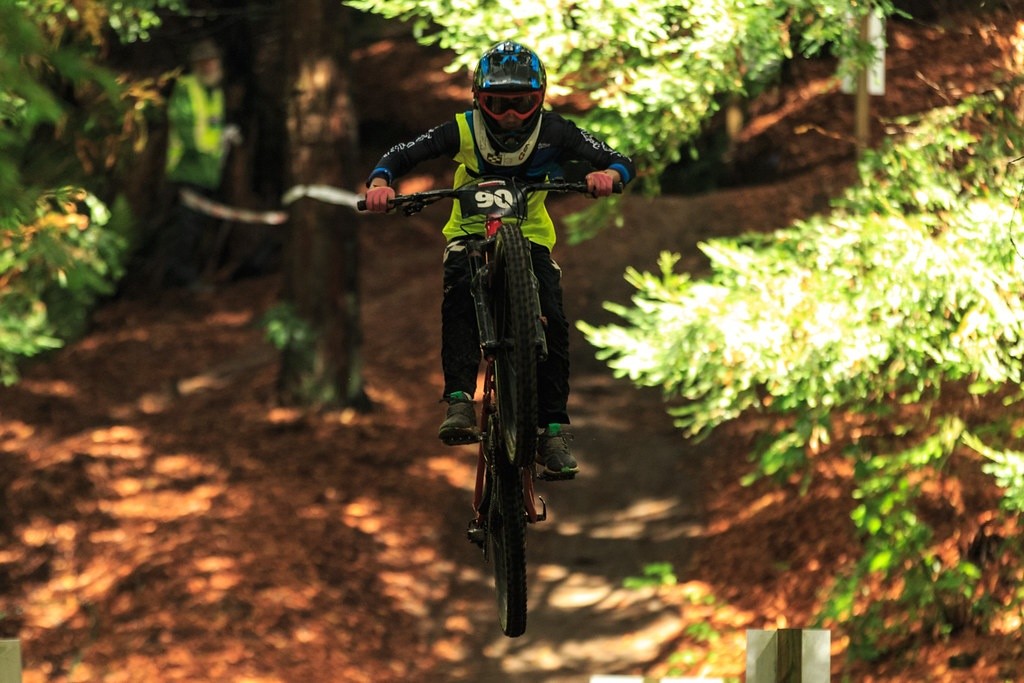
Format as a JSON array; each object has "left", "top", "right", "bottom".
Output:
[
  {"left": 438, "top": 391, "right": 478, "bottom": 439},
  {"left": 535, "top": 422, "right": 580, "bottom": 474}
]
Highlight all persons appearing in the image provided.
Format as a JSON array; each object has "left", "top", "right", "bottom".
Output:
[{"left": 365, "top": 39, "right": 635, "bottom": 473}]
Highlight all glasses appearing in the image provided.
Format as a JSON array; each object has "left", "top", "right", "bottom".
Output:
[{"left": 477, "top": 91, "right": 543, "bottom": 120}]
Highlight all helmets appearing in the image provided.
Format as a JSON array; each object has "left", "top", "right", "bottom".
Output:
[{"left": 471, "top": 38, "right": 546, "bottom": 151}]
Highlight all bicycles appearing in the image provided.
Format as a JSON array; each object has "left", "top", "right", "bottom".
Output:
[{"left": 356, "top": 182, "right": 623, "bottom": 638}]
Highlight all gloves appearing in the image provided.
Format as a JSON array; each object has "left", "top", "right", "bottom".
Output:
[
  {"left": 366, "top": 186, "right": 395, "bottom": 212},
  {"left": 588, "top": 172, "right": 613, "bottom": 197}
]
